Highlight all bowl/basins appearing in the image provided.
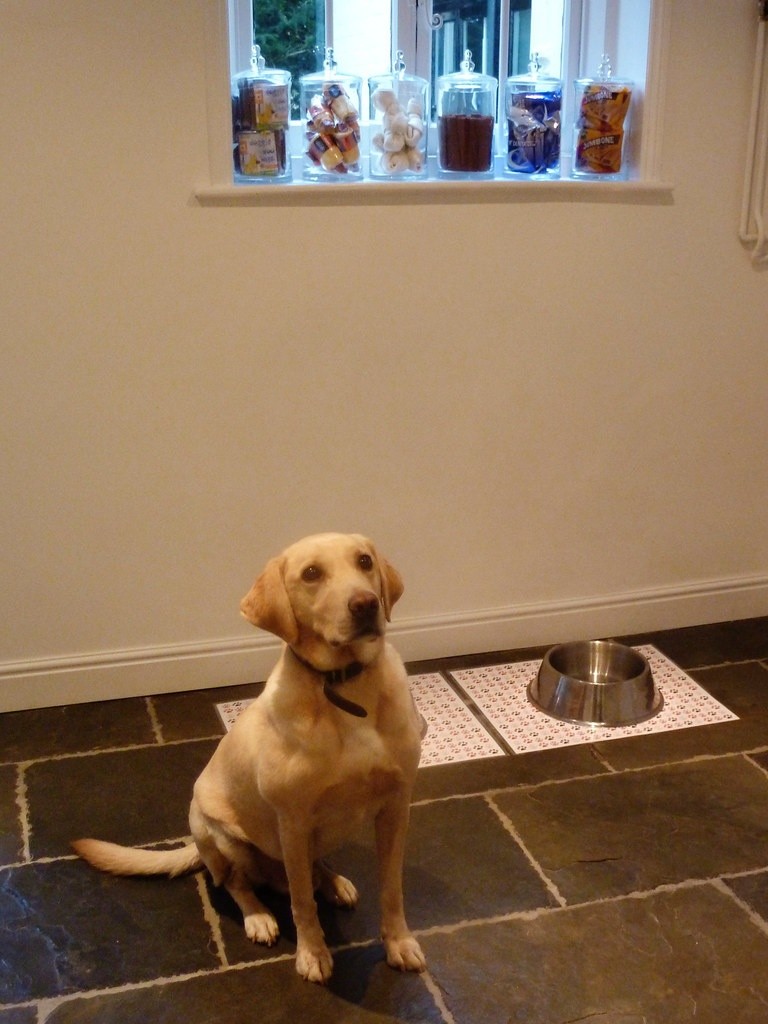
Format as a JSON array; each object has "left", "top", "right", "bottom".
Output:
[{"left": 525, "top": 637, "right": 663, "bottom": 729}]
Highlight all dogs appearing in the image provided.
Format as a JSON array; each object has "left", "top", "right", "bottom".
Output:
[{"left": 69, "top": 532, "right": 430, "bottom": 985}]
